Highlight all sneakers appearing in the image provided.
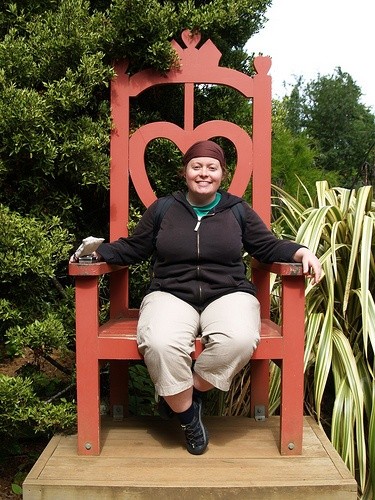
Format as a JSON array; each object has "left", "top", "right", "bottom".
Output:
[
  {"left": 181, "top": 401, "right": 208, "bottom": 455},
  {"left": 159, "top": 404, "right": 178, "bottom": 421}
]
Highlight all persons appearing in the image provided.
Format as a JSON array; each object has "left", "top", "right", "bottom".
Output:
[{"left": 69, "top": 138, "right": 323, "bottom": 455}]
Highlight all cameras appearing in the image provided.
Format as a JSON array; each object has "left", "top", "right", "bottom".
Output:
[{"left": 79, "top": 256, "right": 97, "bottom": 263}]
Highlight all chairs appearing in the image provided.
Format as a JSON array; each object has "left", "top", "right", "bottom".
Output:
[{"left": 67, "top": 30, "right": 312, "bottom": 455}]
